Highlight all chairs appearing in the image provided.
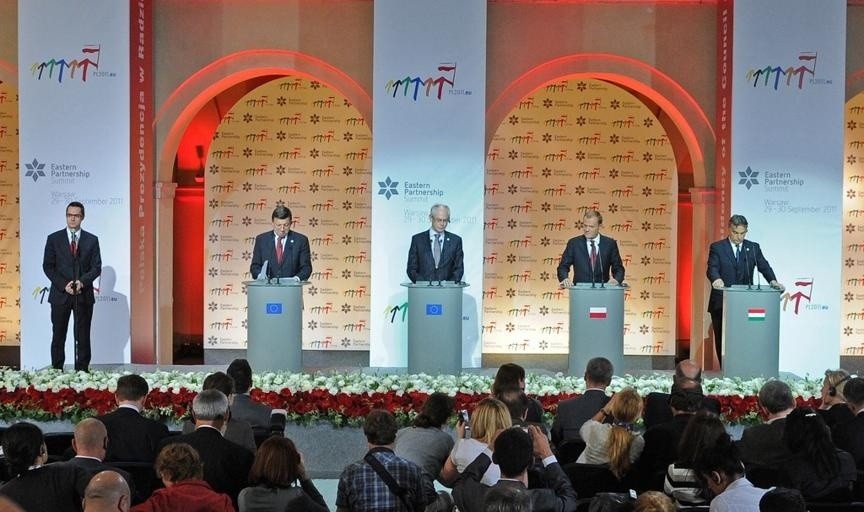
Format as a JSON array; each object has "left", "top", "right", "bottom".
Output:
[
  {"left": 557, "top": 441, "right": 771, "bottom": 500},
  {"left": 0, "top": 428, "right": 154, "bottom": 501}
]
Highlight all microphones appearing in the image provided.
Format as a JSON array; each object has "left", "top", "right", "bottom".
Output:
[
  {"left": 438, "top": 239, "right": 443, "bottom": 286},
  {"left": 751, "top": 244, "right": 763, "bottom": 290},
  {"left": 70, "top": 227, "right": 76, "bottom": 244},
  {"left": 427, "top": 239, "right": 433, "bottom": 286},
  {"left": 589, "top": 243, "right": 595, "bottom": 289},
  {"left": 597, "top": 243, "right": 605, "bottom": 288},
  {"left": 742, "top": 244, "right": 753, "bottom": 290}
]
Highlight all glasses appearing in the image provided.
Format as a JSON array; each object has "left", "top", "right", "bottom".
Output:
[
  {"left": 66, "top": 213, "right": 82, "bottom": 218},
  {"left": 433, "top": 216, "right": 450, "bottom": 224}
]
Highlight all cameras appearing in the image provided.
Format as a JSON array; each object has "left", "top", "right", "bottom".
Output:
[{"left": 267, "top": 408, "right": 288, "bottom": 438}]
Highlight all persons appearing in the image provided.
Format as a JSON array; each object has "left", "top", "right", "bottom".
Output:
[
  {"left": 407, "top": 204, "right": 463, "bottom": 285},
  {"left": 334, "top": 356, "right": 864, "bottom": 511},
  {"left": 42, "top": 201, "right": 101, "bottom": 373},
  {"left": 2, "top": 357, "right": 331, "bottom": 511},
  {"left": 557, "top": 211, "right": 625, "bottom": 286},
  {"left": 250, "top": 205, "right": 313, "bottom": 282},
  {"left": 706, "top": 215, "right": 783, "bottom": 368}
]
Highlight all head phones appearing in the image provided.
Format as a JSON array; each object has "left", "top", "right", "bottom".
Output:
[{"left": 828, "top": 375, "right": 851, "bottom": 396}]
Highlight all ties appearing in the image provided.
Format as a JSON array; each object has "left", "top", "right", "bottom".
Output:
[
  {"left": 275, "top": 236, "right": 285, "bottom": 262},
  {"left": 589, "top": 240, "right": 597, "bottom": 271},
  {"left": 735, "top": 243, "right": 740, "bottom": 262},
  {"left": 70, "top": 233, "right": 78, "bottom": 254},
  {"left": 434, "top": 233, "right": 441, "bottom": 267}
]
